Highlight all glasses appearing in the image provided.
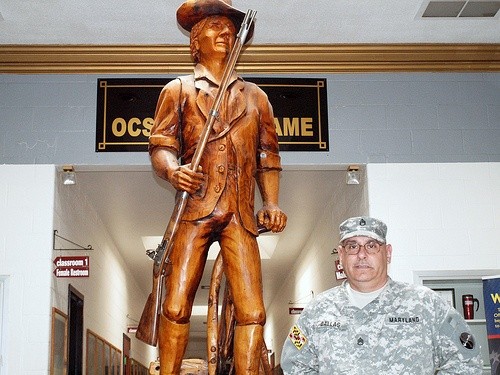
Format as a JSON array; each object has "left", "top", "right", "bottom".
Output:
[{"left": 341, "top": 239, "right": 383, "bottom": 255}]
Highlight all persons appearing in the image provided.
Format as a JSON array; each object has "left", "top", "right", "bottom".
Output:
[
  {"left": 157, "top": 1, "right": 287, "bottom": 375},
  {"left": 280, "top": 218, "right": 483, "bottom": 375}
]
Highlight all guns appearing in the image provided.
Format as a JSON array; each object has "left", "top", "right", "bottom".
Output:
[{"left": 128, "top": 9, "right": 258, "bottom": 347}]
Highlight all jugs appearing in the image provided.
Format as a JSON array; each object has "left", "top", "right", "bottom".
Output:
[{"left": 462, "top": 295, "right": 479, "bottom": 319}]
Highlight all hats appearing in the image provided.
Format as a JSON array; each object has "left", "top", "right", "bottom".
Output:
[{"left": 338, "top": 216, "right": 388, "bottom": 244}]
